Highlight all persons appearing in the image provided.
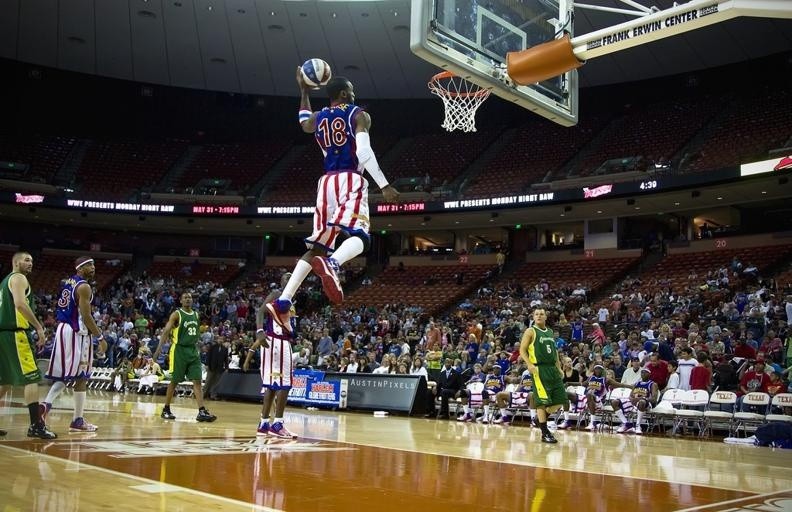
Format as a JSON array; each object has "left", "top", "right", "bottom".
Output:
[
  {"left": 0, "top": 223, "right": 792, "bottom": 451},
  {"left": 265, "top": 65, "right": 402, "bottom": 333}
]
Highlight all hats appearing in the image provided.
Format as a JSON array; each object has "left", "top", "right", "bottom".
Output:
[{"left": 754, "top": 358, "right": 766, "bottom": 367}]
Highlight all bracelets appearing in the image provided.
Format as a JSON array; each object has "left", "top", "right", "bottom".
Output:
[{"left": 298, "top": 107, "right": 313, "bottom": 124}]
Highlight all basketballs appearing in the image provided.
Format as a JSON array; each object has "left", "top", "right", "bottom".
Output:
[{"left": 301, "top": 58, "right": 332, "bottom": 91}]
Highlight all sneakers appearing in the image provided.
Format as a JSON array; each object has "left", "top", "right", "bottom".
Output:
[
  {"left": 268, "top": 421, "right": 298, "bottom": 440},
  {"left": 617, "top": 421, "right": 634, "bottom": 434},
  {"left": 68, "top": 416, "right": 98, "bottom": 433},
  {"left": 635, "top": 425, "right": 643, "bottom": 434},
  {"left": 529, "top": 417, "right": 605, "bottom": 431},
  {"left": 161, "top": 411, "right": 176, "bottom": 419},
  {"left": 541, "top": 434, "right": 558, "bottom": 443},
  {"left": 38, "top": 401, "right": 52, "bottom": 427},
  {"left": 0, "top": 429, "right": 8, "bottom": 438},
  {"left": 311, "top": 255, "right": 345, "bottom": 305},
  {"left": 256, "top": 420, "right": 273, "bottom": 439},
  {"left": 423, "top": 410, "right": 511, "bottom": 425},
  {"left": 264, "top": 294, "right": 298, "bottom": 335},
  {"left": 195, "top": 411, "right": 217, "bottom": 422},
  {"left": 26, "top": 423, "right": 57, "bottom": 440}
]
